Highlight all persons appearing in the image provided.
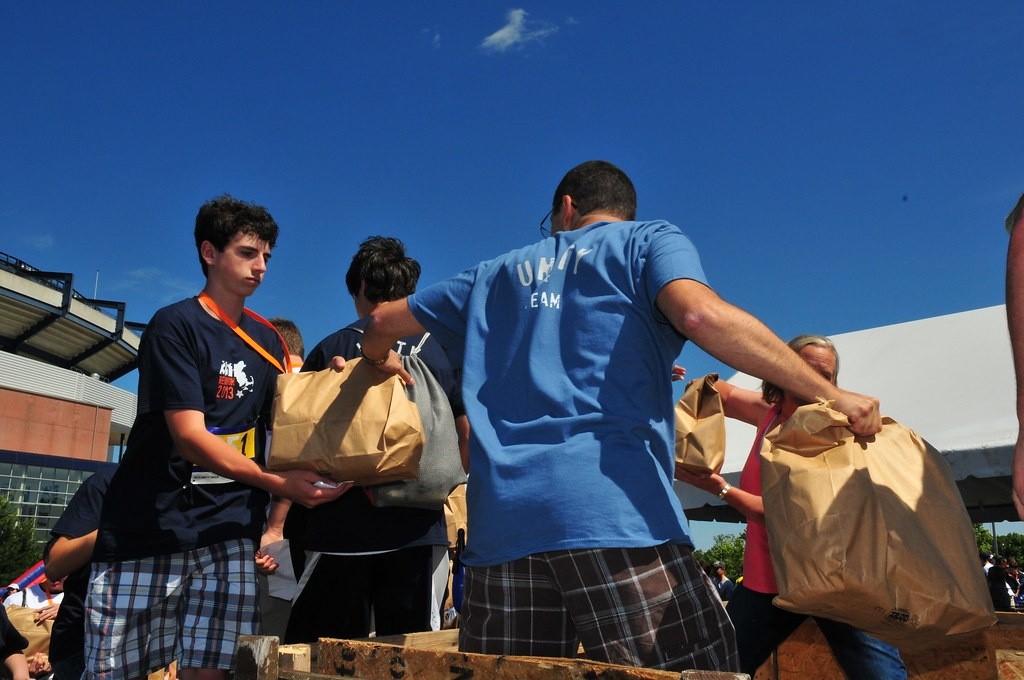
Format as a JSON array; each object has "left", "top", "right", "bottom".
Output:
[
  {"left": 363, "top": 161, "right": 907, "bottom": 680},
  {"left": 43, "top": 195, "right": 470, "bottom": 680},
  {"left": 0, "top": 537, "right": 68, "bottom": 680},
  {"left": 980, "top": 552, "right": 1024, "bottom": 612},
  {"left": 1006, "top": 194, "right": 1024, "bottom": 521}
]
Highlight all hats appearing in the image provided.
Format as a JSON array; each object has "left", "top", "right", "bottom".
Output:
[
  {"left": 7, "top": 583, "right": 20, "bottom": 590},
  {"left": 712, "top": 560, "right": 725, "bottom": 569}
]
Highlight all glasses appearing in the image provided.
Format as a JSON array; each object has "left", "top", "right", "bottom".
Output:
[{"left": 539, "top": 200, "right": 577, "bottom": 239}]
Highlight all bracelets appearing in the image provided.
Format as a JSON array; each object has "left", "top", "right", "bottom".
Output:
[
  {"left": 717, "top": 483, "right": 731, "bottom": 500},
  {"left": 361, "top": 353, "right": 389, "bottom": 366}
]
